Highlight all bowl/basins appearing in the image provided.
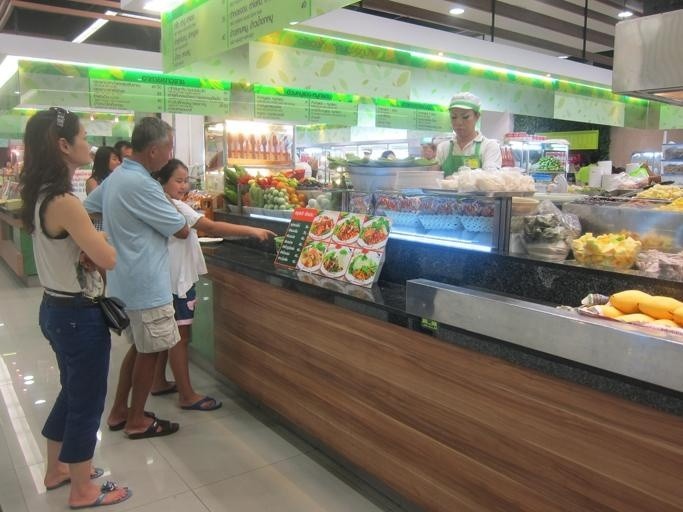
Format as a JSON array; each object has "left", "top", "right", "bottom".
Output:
[
  {"left": 344, "top": 166, "right": 443, "bottom": 192},
  {"left": 510, "top": 197, "right": 540, "bottom": 216}
]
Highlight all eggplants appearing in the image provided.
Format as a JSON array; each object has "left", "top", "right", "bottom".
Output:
[{"left": 224, "top": 164, "right": 247, "bottom": 203}]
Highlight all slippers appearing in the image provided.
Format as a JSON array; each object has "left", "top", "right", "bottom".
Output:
[
  {"left": 128, "top": 416, "right": 179, "bottom": 439},
  {"left": 151, "top": 384, "right": 178, "bottom": 395},
  {"left": 109, "top": 410, "right": 154, "bottom": 431},
  {"left": 181, "top": 396, "right": 221, "bottom": 410},
  {"left": 70, "top": 480, "right": 132, "bottom": 509},
  {"left": 46, "top": 467, "right": 104, "bottom": 490}
]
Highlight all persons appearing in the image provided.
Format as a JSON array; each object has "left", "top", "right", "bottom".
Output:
[
  {"left": 86, "top": 140, "right": 122, "bottom": 232},
  {"left": 114, "top": 140, "right": 133, "bottom": 161},
  {"left": 15, "top": 107, "right": 128, "bottom": 506},
  {"left": 151, "top": 159, "right": 278, "bottom": 408},
  {"left": 83, "top": 116, "right": 189, "bottom": 435},
  {"left": 421, "top": 143, "right": 437, "bottom": 159},
  {"left": 429, "top": 92, "right": 502, "bottom": 180}
]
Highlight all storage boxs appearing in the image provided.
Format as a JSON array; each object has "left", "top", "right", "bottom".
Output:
[
  {"left": 660, "top": 144, "right": 683, "bottom": 185},
  {"left": 350, "top": 165, "right": 445, "bottom": 191}
]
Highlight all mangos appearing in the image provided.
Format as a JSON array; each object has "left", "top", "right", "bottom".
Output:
[{"left": 601, "top": 290, "right": 683, "bottom": 328}]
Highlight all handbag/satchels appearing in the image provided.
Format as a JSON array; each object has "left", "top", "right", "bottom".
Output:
[{"left": 100, "top": 297, "right": 130, "bottom": 336}]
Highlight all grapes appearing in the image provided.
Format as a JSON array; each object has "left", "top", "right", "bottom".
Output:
[{"left": 262, "top": 187, "right": 287, "bottom": 210}]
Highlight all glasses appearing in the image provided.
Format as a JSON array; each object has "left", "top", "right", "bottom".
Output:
[{"left": 50, "top": 106, "right": 69, "bottom": 128}]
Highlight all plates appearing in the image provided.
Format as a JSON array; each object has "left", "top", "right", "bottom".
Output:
[
  {"left": 197, "top": 237, "right": 224, "bottom": 246},
  {"left": 295, "top": 214, "right": 391, "bottom": 285}
]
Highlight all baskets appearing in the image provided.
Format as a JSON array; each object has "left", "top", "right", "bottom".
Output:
[
  {"left": 384, "top": 210, "right": 436, "bottom": 228},
  {"left": 460, "top": 215, "right": 535, "bottom": 233},
  {"left": 418, "top": 215, "right": 462, "bottom": 230}
]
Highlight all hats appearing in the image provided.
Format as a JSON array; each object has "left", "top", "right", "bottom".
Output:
[{"left": 448, "top": 92, "right": 481, "bottom": 113}]
